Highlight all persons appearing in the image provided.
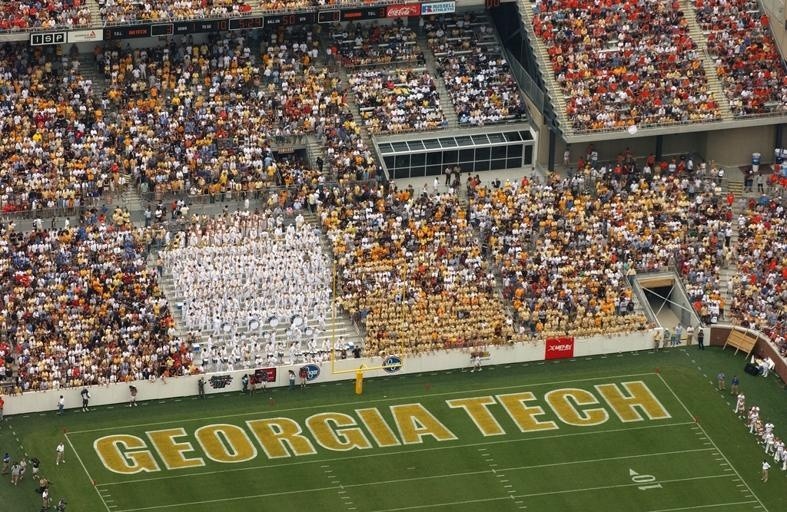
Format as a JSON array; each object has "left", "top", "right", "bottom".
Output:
[
  {"left": 80, "top": 389, "right": 90, "bottom": 413},
  {"left": 126, "top": 385, "right": 138, "bottom": 408},
  {"left": 532, "top": 0, "right": 786, "bottom": 136},
  {"left": 55, "top": 441, "right": 66, "bottom": 465},
  {"left": 1, "top": 13, "right": 527, "bottom": 215},
  {"left": 0, "top": 450, "right": 67, "bottom": 512},
  {"left": 0, "top": 1, "right": 406, "bottom": 34},
  {"left": 56, "top": 395, "right": 64, "bottom": 414},
  {"left": 759, "top": 458, "right": 771, "bottom": 484},
  {"left": 0, "top": 397, "right": 5, "bottom": 423},
  {"left": 0, "top": 143, "right": 786, "bottom": 398},
  {"left": 731, "top": 396, "right": 786, "bottom": 471}
]
